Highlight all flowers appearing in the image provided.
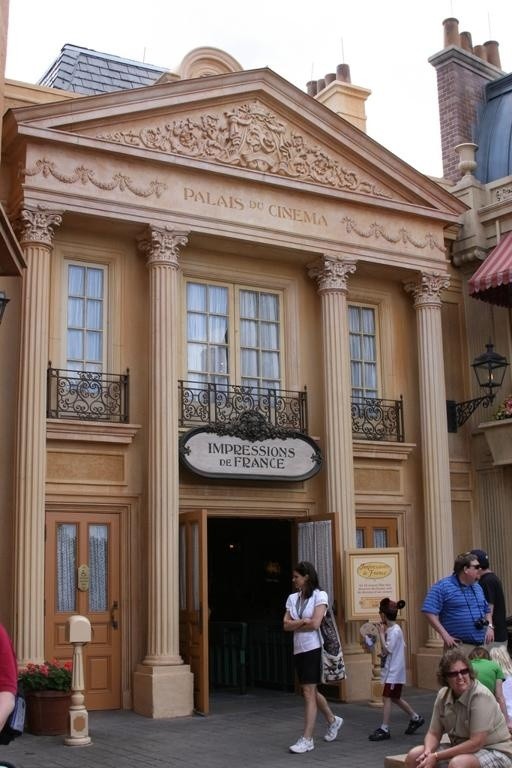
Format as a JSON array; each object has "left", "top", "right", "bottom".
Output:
[
  {"left": 486, "top": 391, "right": 512, "bottom": 422},
  {"left": 13, "top": 655, "right": 76, "bottom": 697}
]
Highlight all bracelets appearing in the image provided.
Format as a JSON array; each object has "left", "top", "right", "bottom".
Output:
[{"left": 435, "top": 751, "right": 440, "bottom": 761}]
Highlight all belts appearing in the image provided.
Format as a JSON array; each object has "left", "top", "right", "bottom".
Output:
[{"left": 455, "top": 640, "right": 484, "bottom": 646}]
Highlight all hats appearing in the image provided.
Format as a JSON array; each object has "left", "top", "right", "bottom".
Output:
[
  {"left": 471, "top": 549, "right": 489, "bottom": 563},
  {"left": 379, "top": 597, "right": 406, "bottom": 613}
]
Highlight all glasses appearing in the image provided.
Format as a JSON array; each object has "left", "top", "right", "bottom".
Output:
[
  {"left": 469, "top": 565, "right": 481, "bottom": 569},
  {"left": 443, "top": 668, "right": 469, "bottom": 678}
]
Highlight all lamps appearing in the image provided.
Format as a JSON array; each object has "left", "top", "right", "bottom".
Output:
[{"left": 444, "top": 332, "right": 511, "bottom": 437}]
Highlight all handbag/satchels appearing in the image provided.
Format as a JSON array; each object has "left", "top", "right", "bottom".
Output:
[
  {"left": 321, "top": 616, "right": 339, "bottom": 656},
  {"left": 321, "top": 646, "right": 348, "bottom": 686}
]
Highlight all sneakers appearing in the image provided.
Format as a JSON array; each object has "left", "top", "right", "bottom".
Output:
[
  {"left": 405, "top": 715, "right": 425, "bottom": 734},
  {"left": 323, "top": 716, "right": 344, "bottom": 741},
  {"left": 289, "top": 736, "right": 314, "bottom": 754},
  {"left": 368, "top": 728, "right": 390, "bottom": 741}
]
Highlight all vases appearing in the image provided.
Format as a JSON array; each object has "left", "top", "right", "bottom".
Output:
[
  {"left": 16, "top": 688, "right": 76, "bottom": 738},
  {"left": 477, "top": 416, "right": 512, "bottom": 466}
]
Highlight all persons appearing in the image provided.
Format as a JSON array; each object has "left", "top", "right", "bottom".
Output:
[
  {"left": 405, "top": 650, "right": 511, "bottom": 768},
  {"left": 283, "top": 560, "right": 345, "bottom": 755},
  {"left": 368, "top": 597, "right": 425, "bottom": 742},
  {"left": 1, "top": 621, "right": 17, "bottom": 739},
  {"left": 422, "top": 547, "right": 512, "bottom": 732}
]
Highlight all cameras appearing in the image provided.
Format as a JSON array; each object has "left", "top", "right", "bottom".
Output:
[{"left": 475, "top": 618, "right": 489, "bottom": 630}]
